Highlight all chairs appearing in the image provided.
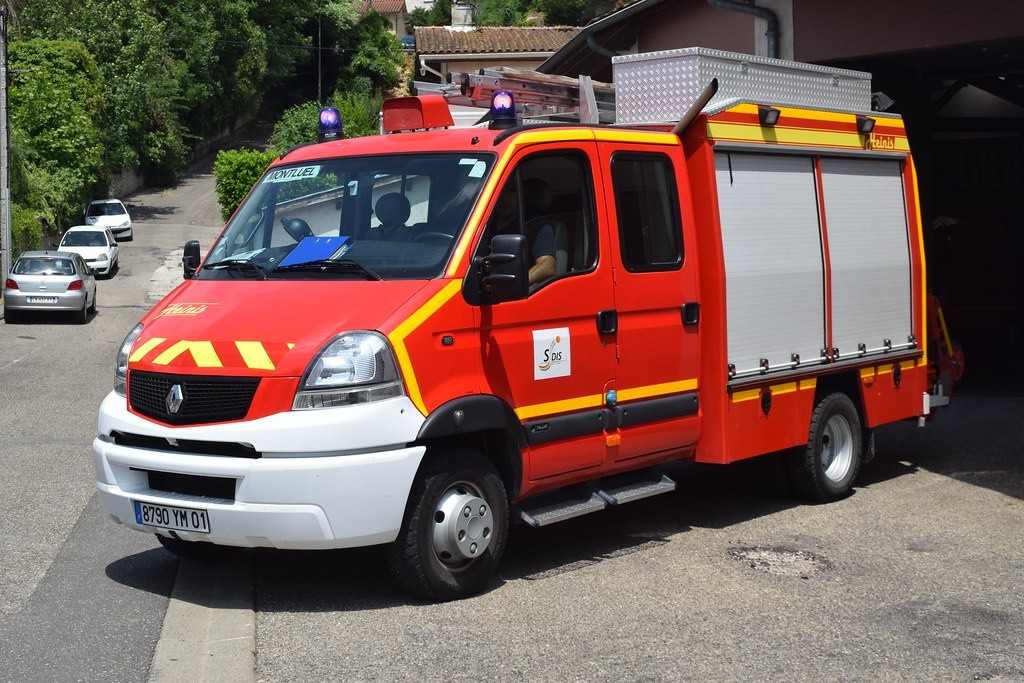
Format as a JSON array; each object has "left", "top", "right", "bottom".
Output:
[
  {"left": 30, "top": 261, "right": 40, "bottom": 269},
  {"left": 362, "top": 192, "right": 420, "bottom": 242},
  {"left": 523, "top": 178, "right": 568, "bottom": 275}
]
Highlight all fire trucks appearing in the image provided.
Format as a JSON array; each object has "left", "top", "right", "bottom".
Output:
[{"left": 90, "top": 43, "right": 968, "bottom": 604}]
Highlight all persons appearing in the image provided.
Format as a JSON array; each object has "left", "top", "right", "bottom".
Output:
[{"left": 468, "top": 168, "right": 557, "bottom": 289}]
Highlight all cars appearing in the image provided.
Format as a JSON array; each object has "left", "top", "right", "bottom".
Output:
[
  {"left": 84, "top": 197, "right": 133, "bottom": 242},
  {"left": 57, "top": 225, "right": 119, "bottom": 279},
  {"left": 3, "top": 250, "right": 95, "bottom": 324}
]
